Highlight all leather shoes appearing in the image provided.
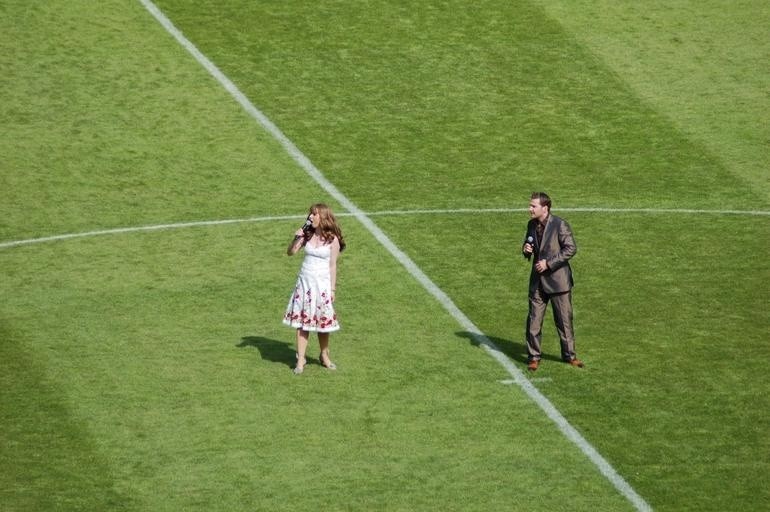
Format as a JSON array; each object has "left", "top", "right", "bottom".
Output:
[
  {"left": 527, "top": 359, "right": 539, "bottom": 372},
  {"left": 566, "top": 358, "right": 583, "bottom": 367}
]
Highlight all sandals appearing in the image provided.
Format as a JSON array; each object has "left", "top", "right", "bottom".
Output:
[
  {"left": 292, "top": 353, "right": 307, "bottom": 375},
  {"left": 319, "top": 349, "right": 336, "bottom": 370}
]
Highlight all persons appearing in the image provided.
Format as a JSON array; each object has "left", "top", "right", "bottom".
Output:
[
  {"left": 522, "top": 192, "right": 584, "bottom": 371},
  {"left": 287, "top": 204, "right": 347, "bottom": 375}
]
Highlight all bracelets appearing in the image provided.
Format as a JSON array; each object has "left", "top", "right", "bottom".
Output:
[{"left": 331, "top": 288, "right": 337, "bottom": 292}]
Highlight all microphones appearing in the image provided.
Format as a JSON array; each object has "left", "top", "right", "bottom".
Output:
[
  {"left": 526, "top": 235, "right": 534, "bottom": 261},
  {"left": 294, "top": 219, "right": 312, "bottom": 241}
]
